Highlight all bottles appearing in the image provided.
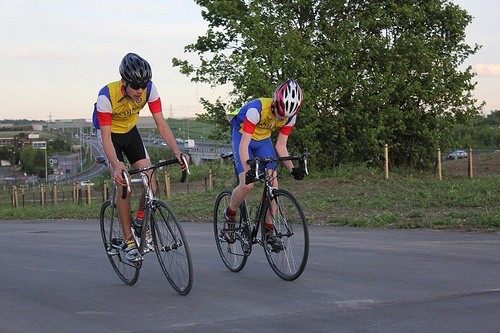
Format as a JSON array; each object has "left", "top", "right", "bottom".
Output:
[{"left": 134, "top": 210, "right": 144, "bottom": 238}]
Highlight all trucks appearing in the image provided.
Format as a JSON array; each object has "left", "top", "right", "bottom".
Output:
[{"left": 183, "top": 139, "right": 194, "bottom": 149}]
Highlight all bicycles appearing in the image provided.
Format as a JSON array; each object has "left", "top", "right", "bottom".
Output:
[
  {"left": 213, "top": 152, "right": 310, "bottom": 282},
  {"left": 100, "top": 153, "right": 197, "bottom": 298}
]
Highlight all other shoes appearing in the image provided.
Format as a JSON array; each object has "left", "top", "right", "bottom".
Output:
[
  {"left": 133, "top": 218, "right": 154, "bottom": 250},
  {"left": 122, "top": 235, "right": 144, "bottom": 262}
]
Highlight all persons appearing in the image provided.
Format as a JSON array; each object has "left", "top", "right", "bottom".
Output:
[
  {"left": 92, "top": 52, "right": 190, "bottom": 263},
  {"left": 223, "top": 80, "right": 304, "bottom": 249}
]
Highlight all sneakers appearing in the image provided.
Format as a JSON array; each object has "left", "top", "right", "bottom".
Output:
[
  {"left": 223, "top": 208, "right": 236, "bottom": 244},
  {"left": 263, "top": 233, "right": 288, "bottom": 250}
]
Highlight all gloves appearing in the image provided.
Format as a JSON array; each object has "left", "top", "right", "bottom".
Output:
[
  {"left": 244, "top": 170, "right": 255, "bottom": 184},
  {"left": 291, "top": 167, "right": 306, "bottom": 181}
]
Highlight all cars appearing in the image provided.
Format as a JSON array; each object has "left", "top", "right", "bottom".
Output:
[
  {"left": 80, "top": 181, "right": 95, "bottom": 186},
  {"left": 448, "top": 150, "right": 468, "bottom": 160},
  {"left": 152, "top": 137, "right": 183, "bottom": 146}
]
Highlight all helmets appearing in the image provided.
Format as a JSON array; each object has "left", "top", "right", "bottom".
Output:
[
  {"left": 273, "top": 78, "right": 304, "bottom": 118},
  {"left": 119, "top": 52, "right": 152, "bottom": 84}
]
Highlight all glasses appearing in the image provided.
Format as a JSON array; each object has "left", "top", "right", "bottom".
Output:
[{"left": 130, "top": 80, "right": 150, "bottom": 90}]
